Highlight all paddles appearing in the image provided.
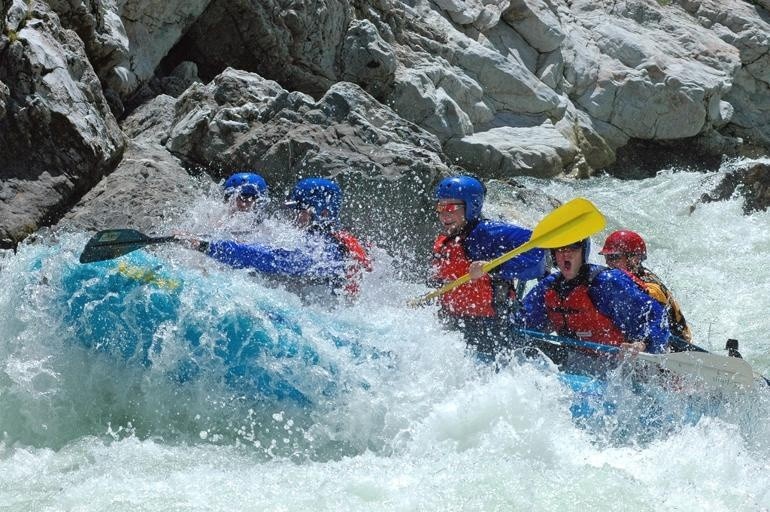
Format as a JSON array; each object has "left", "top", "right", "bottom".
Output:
[
  {"left": 79, "top": 229, "right": 174, "bottom": 264},
  {"left": 515, "top": 333, "right": 757, "bottom": 392},
  {"left": 414, "top": 198, "right": 609, "bottom": 310}
]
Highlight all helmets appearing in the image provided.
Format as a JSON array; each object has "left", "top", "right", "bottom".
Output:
[
  {"left": 550, "top": 236, "right": 591, "bottom": 265},
  {"left": 599, "top": 230, "right": 647, "bottom": 260},
  {"left": 285, "top": 179, "right": 342, "bottom": 221},
  {"left": 432, "top": 175, "right": 484, "bottom": 220},
  {"left": 223, "top": 172, "right": 268, "bottom": 198}
]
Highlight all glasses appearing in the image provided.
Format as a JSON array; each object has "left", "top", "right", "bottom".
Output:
[{"left": 436, "top": 203, "right": 464, "bottom": 213}]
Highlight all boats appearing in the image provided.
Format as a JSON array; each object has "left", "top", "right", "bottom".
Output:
[{"left": 22, "top": 242, "right": 766, "bottom": 441}]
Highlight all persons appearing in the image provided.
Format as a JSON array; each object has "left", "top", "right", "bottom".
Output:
[
  {"left": 522, "top": 237, "right": 663, "bottom": 377},
  {"left": 221, "top": 173, "right": 267, "bottom": 223},
  {"left": 176, "top": 180, "right": 371, "bottom": 292},
  {"left": 599, "top": 231, "right": 693, "bottom": 353},
  {"left": 408, "top": 175, "right": 545, "bottom": 352}
]
